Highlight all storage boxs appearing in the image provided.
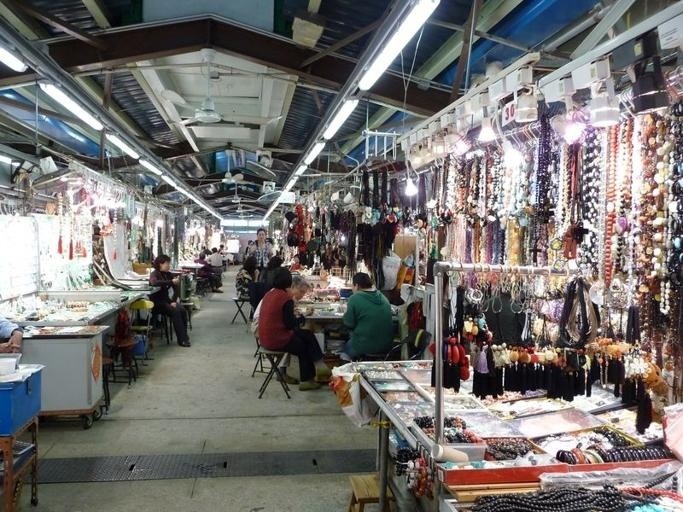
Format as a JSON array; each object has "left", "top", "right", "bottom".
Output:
[{"left": 0, "top": 366, "right": 43, "bottom": 435}]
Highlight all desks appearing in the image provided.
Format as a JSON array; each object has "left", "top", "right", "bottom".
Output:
[
  {"left": 0, "top": 415, "right": 45, "bottom": 511},
  {"left": 294, "top": 300, "right": 360, "bottom": 322},
  {"left": 0, "top": 289, "right": 143, "bottom": 409},
  {"left": 352, "top": 357, "right": 683, "bottom": 512}
]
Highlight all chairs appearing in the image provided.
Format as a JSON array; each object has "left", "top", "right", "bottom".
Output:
[{"left": 384, "top": 328, "right": 432, "bottom": 362}]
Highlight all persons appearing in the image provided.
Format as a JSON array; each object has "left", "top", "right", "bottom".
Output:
[
  {"left": 341, "top": 270, "right": 396, "bottom": 363},
  {"left": 0, "top": 315, "right": 25, "bottom": 353},
  {"left": 250, "top": 275, "right": 310, "bottom": 385},
  {"left": 554, "top": 445, "right": 669, "bottom": 466},
  {"left": 257, "top": 268, "right": 331, "bottom": 391},
  {"left": 147, "top": 254, "right": 192, "bottom": 348},
  {"left": 191, "top": 228, "right": 283, "bottom": 320}
]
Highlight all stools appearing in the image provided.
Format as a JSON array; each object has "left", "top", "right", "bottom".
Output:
[
  {"left": 229, "top": 297, "right": 251, "bottom": 325},
  {"left": 102, "top": 293, "right": 204, "bottom": 408},
  {"left": 344, "top": 469, "right": 394, "bottom": 512},
  {"left": 256, "top": 345, "right": 290, "bottom": 400},
  {"left": 252, "top": 338, "right": 265, "bottom": 378}
]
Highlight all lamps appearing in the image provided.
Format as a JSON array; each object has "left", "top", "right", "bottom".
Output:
[
  {"left": 357, "top": 1, "right": 441, "bottom": 92},
  {"left": 1, "top": 39, "right": 30, "bottom": 74},
  {"left": 35, "top": 76, "right": 105, "bottom": 132},
  {"left": 323, "top": 98, "right": 358, "bottom": 142},
  {"left": 398, "top": 61, "right": 671, "bottom": 196},
  {"left": 287, "top": 142, "right": 324, "bottom": 193},
  {"left": 106, "top": 120, "right": 178, "bottom": 210}
]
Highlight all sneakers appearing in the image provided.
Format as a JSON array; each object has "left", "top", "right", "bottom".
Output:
[
  {"left": 276, "top": 372, "right": 300, "bottom": 385},
  {"left": 212, "top": 287, "right": 224, "bottom": 293},
  {"left": 178, "top": 338, "right": 191, "bottom": 348},
  {"left": 315, "top": 367, "right": 333, "bottom": 379},
  {"left": 298, "top": 381, "right": 322, "bottom": 392}
]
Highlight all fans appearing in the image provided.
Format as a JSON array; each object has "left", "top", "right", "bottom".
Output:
[
  {"left": 12, "top": 152, "right": 76, "bottom": 196},
  {"left": 161, "top": 46, "right": 281, "bottom": 132},
  {"left": 254, "top": 180, "right": 283, "bottom": 204},
  {"left": 178, "top": 146, "right": 257, "bottom": 218},
  {"left": 246, "top": 148, "right": 277, "bottom": 178}
]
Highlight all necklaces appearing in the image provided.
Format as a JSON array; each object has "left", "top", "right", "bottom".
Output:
[{"left": 472, "top": 483, "right": 682, "bottom": 512}]
